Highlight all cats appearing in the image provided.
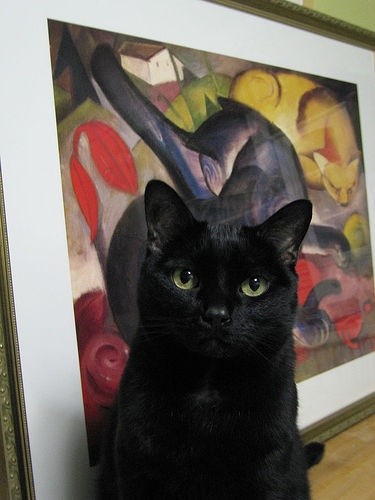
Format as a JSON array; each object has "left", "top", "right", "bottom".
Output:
[{"left": 96, "top": 179, "right": 327, "bottom": 500}]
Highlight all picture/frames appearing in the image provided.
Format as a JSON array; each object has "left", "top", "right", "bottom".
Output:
[{"left": 0, "top": 0, "right": 375, "bottom": 500}]
